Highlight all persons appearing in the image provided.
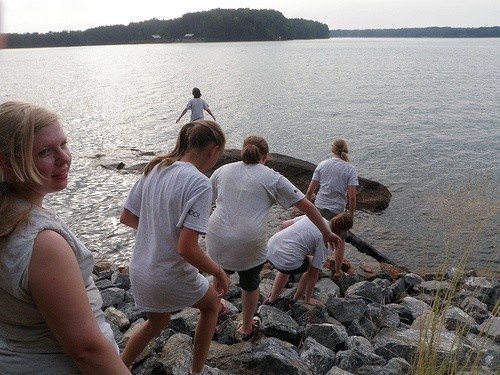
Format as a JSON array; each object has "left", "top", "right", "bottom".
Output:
[
  {"left": 209, "top": 135, "right": 343, "bottom": 343},
  {"left": 176, "top": 87, "right": 216, "bottom": 123},
  {"left": 305, "top": 138, "right": 359, "bottom": 278},
  {"left": 119, "top": 120, "right": 229, "bottom": 375},
  {"left": 267, "top": 213, "right": 353, "bottom": 309},
  {"left": 0, "top": 102, "right": 123, "bottom": 375}
]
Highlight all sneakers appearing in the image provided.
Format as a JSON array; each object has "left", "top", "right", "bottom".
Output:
[
  {"left": 190, "top": 365, "right": 219, "bottom": 375},
  {"left": 241, "top": 317, "right": 261, "bottom": 342}
]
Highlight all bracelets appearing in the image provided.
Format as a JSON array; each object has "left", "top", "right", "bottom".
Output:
[
  {"left": 214, "top": 265, "right": 220, "bottom": 276},
  {"left": 323, "top": 231, "right": 330, "bottom": 236}
]
